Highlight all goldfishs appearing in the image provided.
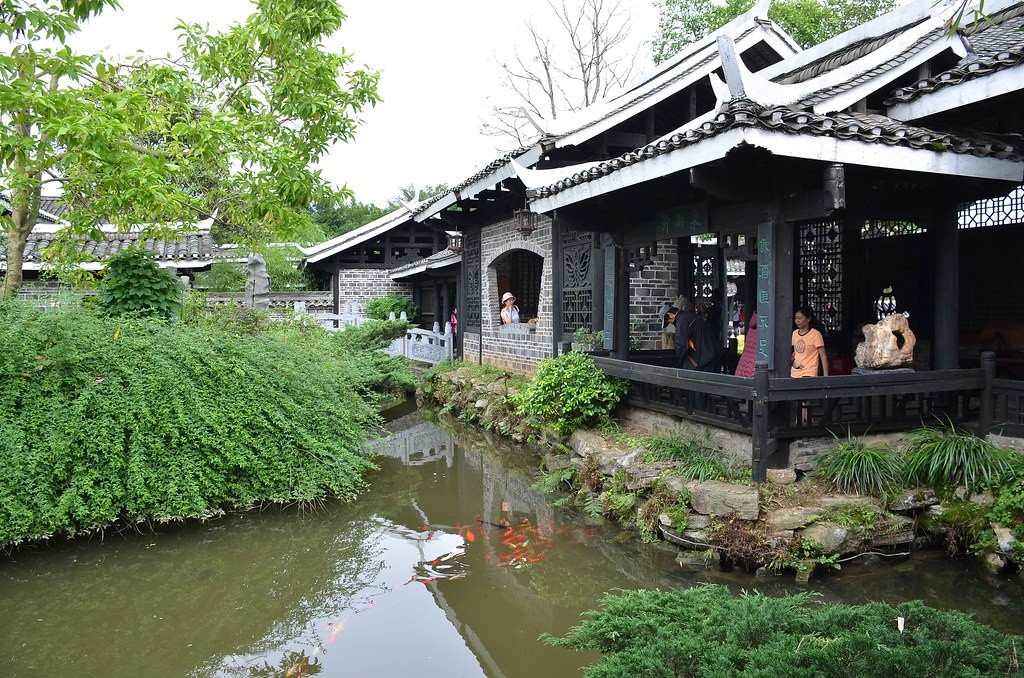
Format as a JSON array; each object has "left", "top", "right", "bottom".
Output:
[
  {"left": 394, "top": 498, "right": 603, "bottom": 590},
  {"left": 282, "top": 591, "right": 381, "bottom": 678}
]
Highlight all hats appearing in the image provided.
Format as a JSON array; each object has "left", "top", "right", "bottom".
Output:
[
  {"left": 502, "top": 292, "right": 516, "bottom": 304},
  {"left": 659, "top": 305, "right": 672, "bottom": 328}
]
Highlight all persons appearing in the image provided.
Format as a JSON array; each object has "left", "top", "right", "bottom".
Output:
[
  {"left": 501, "top": 292, "right": 520, "bottom": 325},
  {"left": 791, "top": 305, "right": 829, "bottom": 424},
  {"left": 658, "top": 304, "right": 722, "bottom": 374},
  {"left": 735, "top": 311, "right": 757, "bottom": 378},
  {"left": 451, "top": 307, "right": 459, "bottom": 352},
  {"left": 727, "top": 304, "right": 745, "bottom": 355}
]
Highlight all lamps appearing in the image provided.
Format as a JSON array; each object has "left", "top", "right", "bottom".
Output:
[
  {"left": 446, "top": 227, "right": 467, "bottom": 254},
  {"left": 514, "top": 199, "right": 539, "bottom": 239}
]
